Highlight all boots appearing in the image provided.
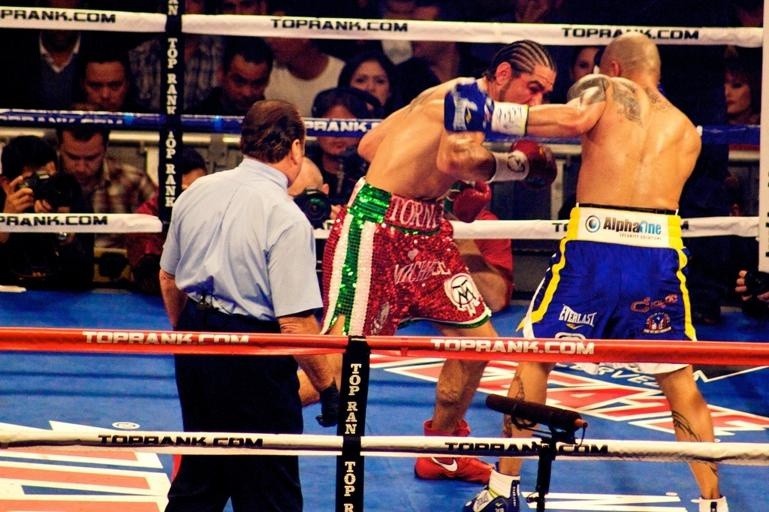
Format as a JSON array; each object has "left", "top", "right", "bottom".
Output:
[
  {"left": 462, "top": 461, "right": 521, "bottom": 512},
  {"left": 698, "top": 493, "right": 729, "bottom": 511},
  {"left": 413, "top": 418, "right": 496, "bottom": 484}
]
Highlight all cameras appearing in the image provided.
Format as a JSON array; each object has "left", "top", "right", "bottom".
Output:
[
  {"left": 745, "top": 271, "right": 769, "bottom": 293},
  {"left": 293, "top": 190, "right": 332, "bottom": 225},
  {"left": 16, "top": 171, "right": 81, "bottom": 205}
]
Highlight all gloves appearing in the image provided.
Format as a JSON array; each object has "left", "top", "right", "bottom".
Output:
[
  {"left": 443, "top": 79, "right": 531, "bottom": 140},
  {"left": 485, "top": 137, "right": 557, "bottom": 191},
  {"left": 442, "top": 178, "right": 493, "bottom": 224},
  {"left": 315, "top": 377, "right": 340, "bottom": 428}
]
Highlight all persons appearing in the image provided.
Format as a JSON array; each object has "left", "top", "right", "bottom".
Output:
[
  {"left": 171, "top": 39, "right": 558, "bottom": 485},
  {"left": 444, "top": 32, "right": 732, "bottom": 512},
  {"left": 159, "top": 99, "right": 341, "bottom": 512}
]
